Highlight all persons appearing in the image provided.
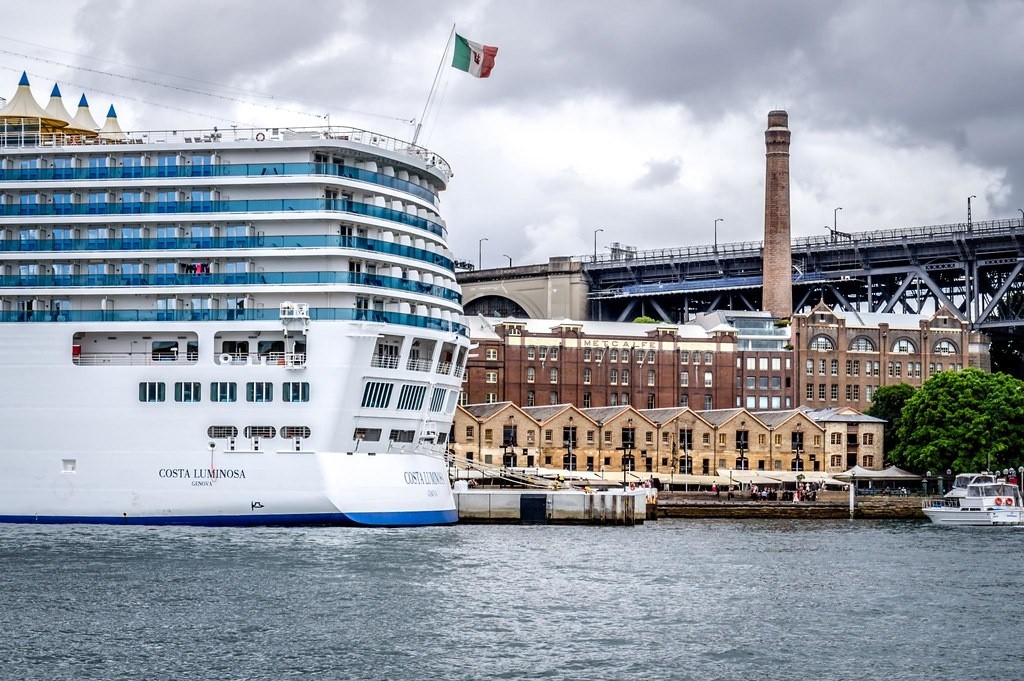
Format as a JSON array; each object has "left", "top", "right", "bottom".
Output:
[{"left": 464, "top": 473, "right": 947, "bottom": 501}]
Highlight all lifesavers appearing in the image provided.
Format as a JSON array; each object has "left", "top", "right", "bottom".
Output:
[
  {"left": 256, "top": 133, "right": 265, "bottom": 141},
  {"left": 1006, "top": 498, "right": 1013, "bottom": 506},
  {"left": 995, "top": 498, "right": 1002, "bottom": 506}
]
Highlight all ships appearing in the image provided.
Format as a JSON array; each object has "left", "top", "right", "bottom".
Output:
[{"left": 0, "top": 113, "right": 472, "bottom": 529}]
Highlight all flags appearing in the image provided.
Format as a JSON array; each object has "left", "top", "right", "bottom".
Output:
[{"left": 451, "top": 32, "right": 499, "bottom": 81}]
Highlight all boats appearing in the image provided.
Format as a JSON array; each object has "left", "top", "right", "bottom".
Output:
[{"left": 922, "top": 471, "right": 1024, "bottom": 530}]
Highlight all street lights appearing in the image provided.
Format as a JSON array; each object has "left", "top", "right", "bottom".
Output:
[
  {"left": 593, "top": 228, "right": 604, "bottom": 261},
  {"left": 834, "top": 205, "right": 842, "bottom": 242},
  {"left": 714, "top": 217, "right": 724, "bottom": 252},
  {"left": 967, "top": 195, "right": 978, "bottom": 232},
  {"left": 478, "top": 237, "right": 490, "bottom": 269},
  {"left": 825, "top": 225, "right": 833, "bottom": 243},
  {"left": 504, "top": 253, "right": 513, "bottom": 267},
  {"left": 507, "top": 414, "right": 856, "bottom": 500}
]
